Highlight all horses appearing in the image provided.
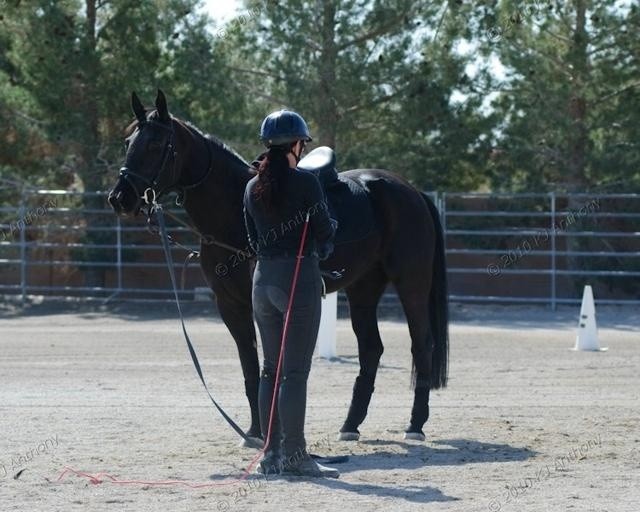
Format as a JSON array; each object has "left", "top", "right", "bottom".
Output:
[{"left": 106, "top": 87, "right": 452, "bottom": 442}]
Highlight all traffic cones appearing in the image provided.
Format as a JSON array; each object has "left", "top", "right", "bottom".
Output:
[{"left": 568, "top": 284, "right": 611, "bottom": 356}]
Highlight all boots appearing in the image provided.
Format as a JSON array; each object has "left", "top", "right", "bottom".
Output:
[
  {"left": 261, "top": 427, "right": 281, "bottom": 474},
  {"left": 279, "top": 425, "right": 341, "bottom": 479}
]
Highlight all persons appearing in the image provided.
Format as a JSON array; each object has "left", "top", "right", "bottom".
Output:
[{"left": 241, "top": 109, "right": 341, "bottom": 478}]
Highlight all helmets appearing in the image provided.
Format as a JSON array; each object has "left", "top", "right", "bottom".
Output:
[{"left": 260, "top": 108, "right": 313, "bottom": 149}]
